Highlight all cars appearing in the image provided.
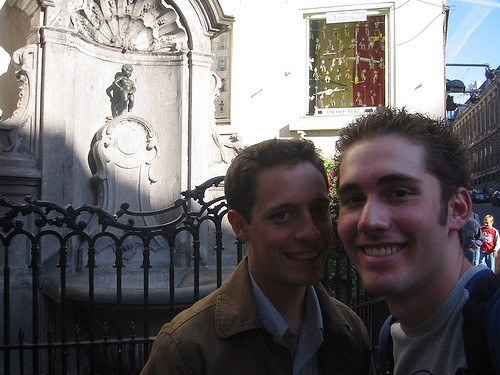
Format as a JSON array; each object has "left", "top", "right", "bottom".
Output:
[
  {"left": 472, "top": 188, "right": 492, "bottom": 204},
  {"left": 493, "top": 189, "right": 500, "bottom": 206}
]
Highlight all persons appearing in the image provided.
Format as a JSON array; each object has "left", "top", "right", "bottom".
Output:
[
  {"left": 463, "top": 217, "right": 484, "bottom": 268},
  {"left": 140, "top": 138, "right": 376, "bottom": 375},
  {"left": 114, "top": 64, "right": 136, "bottom": 116},
  {"left": 334, "top": 107, "right": 500, "bottom": 375},
  {"left": 470, "top": 212, "right": 480, "bottom": 224},
  {"left": 489, "top": 186, "right": 494, "bottom": 198},
  {"left": 479, "top": 215, "right": 500, "bottom": 274}
]
